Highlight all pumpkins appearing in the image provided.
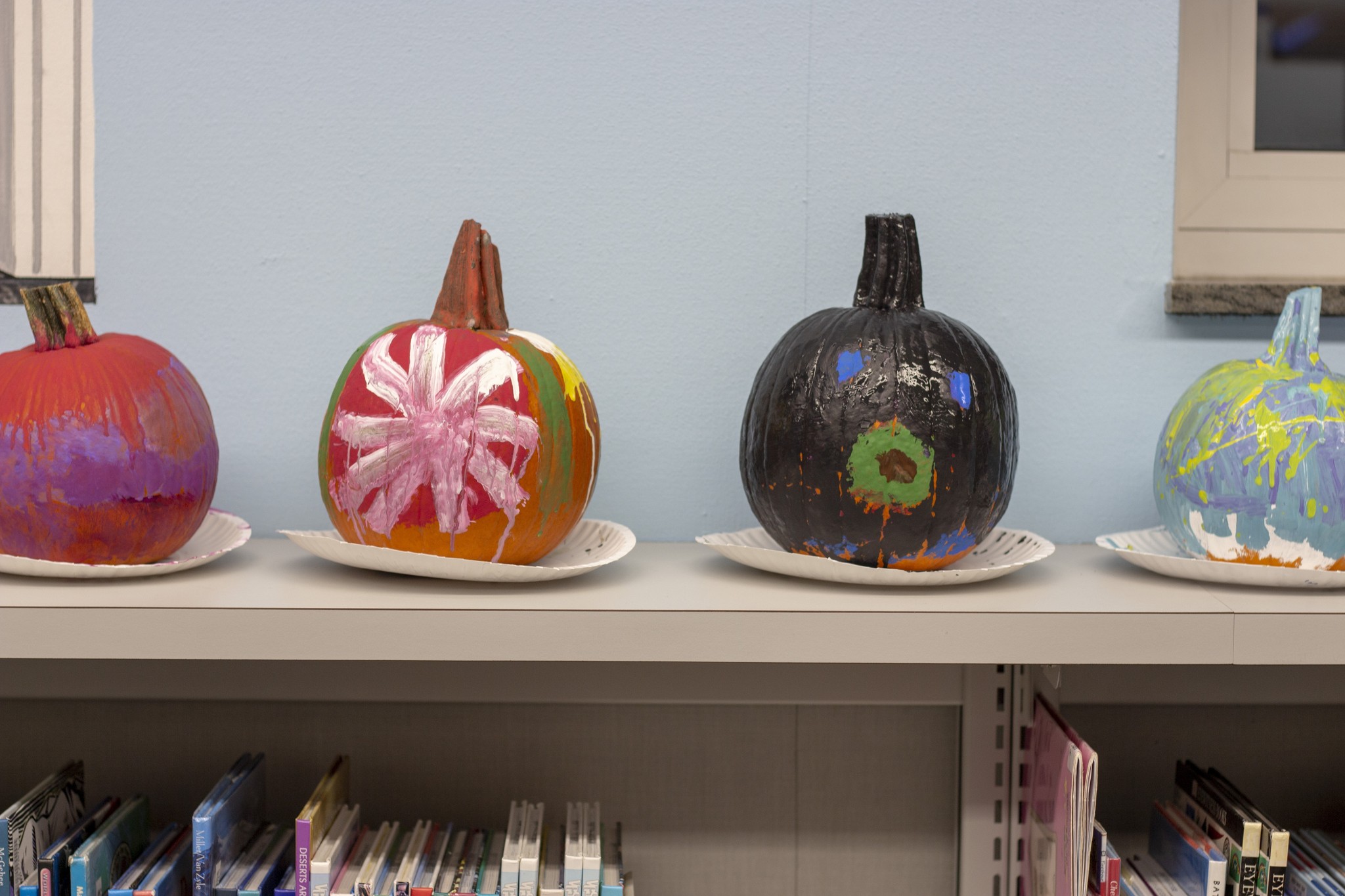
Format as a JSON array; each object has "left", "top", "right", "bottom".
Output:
[
  {"left": 316, "top": 218, "right": 600, "bottom": 566},
  {"left": 0, "top": 281, "right": 220, "bottom": 567},
  {"left": 739, "top": 214, "right": 1020, "bottom": 572},
  {"left": 1152, "top": 287, "right": 1345, "bottom": 571}
]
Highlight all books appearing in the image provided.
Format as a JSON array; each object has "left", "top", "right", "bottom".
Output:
[
  {"left": 0, "top": 749, "right": 634, "bottom": 896},
  {"left": 1019, "top": 688, "right": 1345, "bottom": 896}
]
